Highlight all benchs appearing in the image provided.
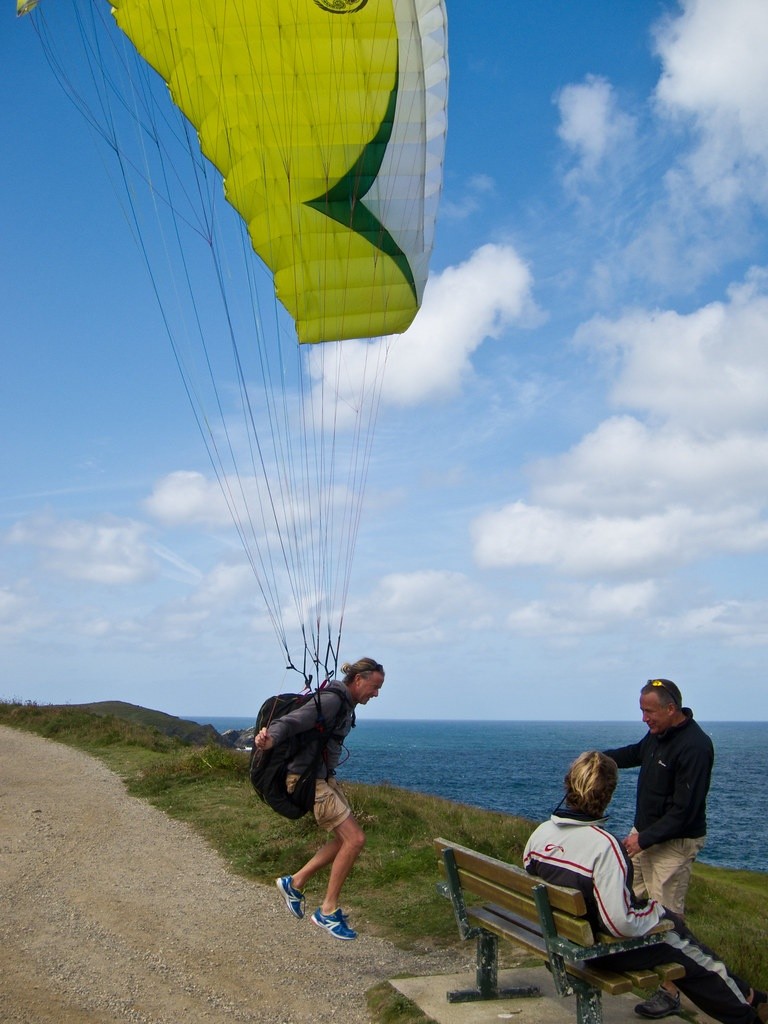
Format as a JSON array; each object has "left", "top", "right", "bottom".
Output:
[{"left": 433, "top": 836, "right": 687, "bottom": 1024}]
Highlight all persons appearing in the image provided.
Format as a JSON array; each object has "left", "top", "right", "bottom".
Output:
[
  {"left": 255, "top": 658, "right": 385, "bottom": 939},
  {"left": 523, "top": 750, "right": 768, "bottom": 1023},
  {"left": 565, "top": 679, "right": 714, "bottom": 1018}
]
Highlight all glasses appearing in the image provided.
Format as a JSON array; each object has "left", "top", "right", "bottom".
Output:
[{"left": 646, "top": 680, "right": 677, "bottom": 705}]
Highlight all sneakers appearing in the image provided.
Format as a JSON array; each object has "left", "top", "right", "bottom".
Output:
[
  {"left": 275, "top": 873, "right": 307, "bottom": 919},
  {"left": 310, "top": 907, "right": 359, "bottom": 940},
  {"left": 634, "top": 985, "right": 682, "bottom": 1019}
]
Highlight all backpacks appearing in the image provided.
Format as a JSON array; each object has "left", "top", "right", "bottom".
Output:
[{"left": 249, "top": 694, "right": 316, "bottom": 820}]
